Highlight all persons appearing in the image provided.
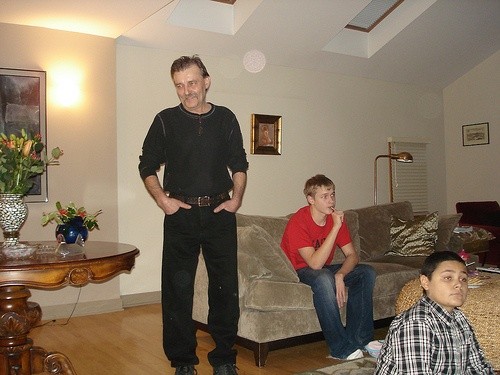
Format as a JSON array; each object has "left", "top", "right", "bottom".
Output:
[
  {"left": 280, "top": 174, "right": 377, "bottom": 360},
  {"left": 138, "top": 54, "right": 248, "bottom": 375},
  {"left": 374, "top": 251, "right": 495, "bottom": 375}
]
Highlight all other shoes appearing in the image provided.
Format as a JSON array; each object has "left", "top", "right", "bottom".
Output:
[
  {"left": 213, "top": 362, "right": 240, "bottom": 375},
  {"left": 175, "top": 360, "right": 198, "bottom": 375}
]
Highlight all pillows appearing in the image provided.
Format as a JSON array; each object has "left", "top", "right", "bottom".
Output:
[
  {"left": 238, "top": 225, "right": 299, "bottom": 283},
  {"left": 384, "top": 211, "right": 438, "bottom": 256},
  {"left": 437, "top": 213, "right": 463, "bottom": 245}
]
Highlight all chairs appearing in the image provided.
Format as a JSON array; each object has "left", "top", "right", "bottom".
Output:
[{"left": 456, "top": 201, "right": 500, "bottom": 266}]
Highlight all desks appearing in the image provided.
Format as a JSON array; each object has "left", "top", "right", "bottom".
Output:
[{"left": 0, "top": 242, "right": 138, "bottom": 375}]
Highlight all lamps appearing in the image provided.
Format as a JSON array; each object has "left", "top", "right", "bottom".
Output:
[{"left": 373, "top": 152, "right": 412, "bottom": 206}]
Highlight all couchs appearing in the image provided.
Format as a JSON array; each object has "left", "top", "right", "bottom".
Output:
[{"left": 192, "top": 203, "right": 465, "bottom": 366}]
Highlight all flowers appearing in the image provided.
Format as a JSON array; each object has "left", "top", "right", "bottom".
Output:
[
  {"left": 0, "top": 129, "right": 64, "bottom": 194},
  {"left": 41, "top": 201, "right": 102, "bottom": 230}
]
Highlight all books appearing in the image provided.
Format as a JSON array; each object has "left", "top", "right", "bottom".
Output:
[{"left": 453, "top": 226, "right": 472, "bottom": 233}]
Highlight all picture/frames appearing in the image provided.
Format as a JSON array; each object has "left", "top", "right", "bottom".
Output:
[
  {"left": 462, "top": 123, "right": 490, "bottom": 147},
  {"left": 250, "top": 114, "right": 282, "bottom": 155},
  {"left": 0, "top": 68, "right": 48, "bottom": 202}
]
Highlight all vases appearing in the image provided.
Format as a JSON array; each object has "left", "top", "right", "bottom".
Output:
[
  {"left": 55, "top": 218, "right": 88, "bottom": 244},
  {"left": 0, "top": 193, "right": 28, "bottom": 248}
]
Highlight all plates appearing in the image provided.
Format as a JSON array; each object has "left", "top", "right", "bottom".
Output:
[{"left": 1, "top": 246, "right": 36, "bottom": 257}]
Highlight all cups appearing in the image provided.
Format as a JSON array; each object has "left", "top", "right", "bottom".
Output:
[{"left": 457, "top": 252, "right": 469, "bottom": 265}]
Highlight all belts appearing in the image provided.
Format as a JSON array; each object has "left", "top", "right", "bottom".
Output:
[{"left": 169, "top": 191, "right": 229, "bottom": 207}]
